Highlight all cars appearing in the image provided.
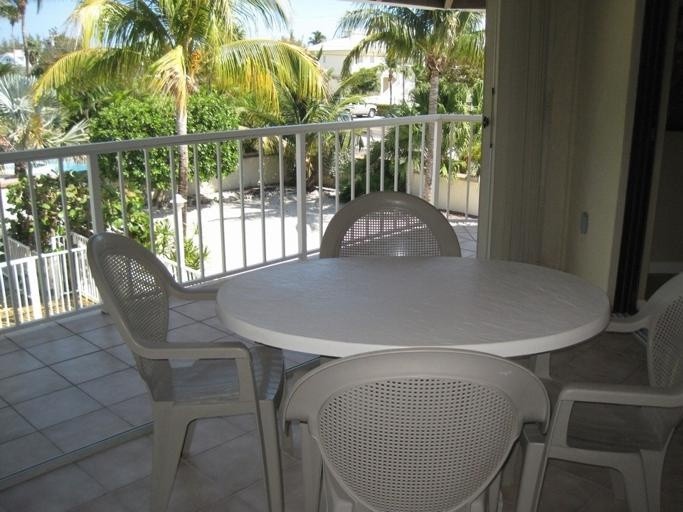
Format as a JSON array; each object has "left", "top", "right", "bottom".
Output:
[{"left": 339, "top": 98, "right": 378, "bottom": 120}]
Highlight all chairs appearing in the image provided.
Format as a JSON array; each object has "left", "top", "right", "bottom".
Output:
[
  {"left": 529, "top": 272, "right": 683, "bottom": 511},
  {"left": 283, "top": 347, "right": 554, "bottom": 512},
  {"left": 320, "top": 190, "right": 462, "bottom": 259},
  {"left": 87, "top": 232, "right": 286, "bottom": 510}
]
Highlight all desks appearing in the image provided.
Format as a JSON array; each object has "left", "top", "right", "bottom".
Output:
[{"left": 213, "top": 256, "right": 614, "bottom": 360}]
